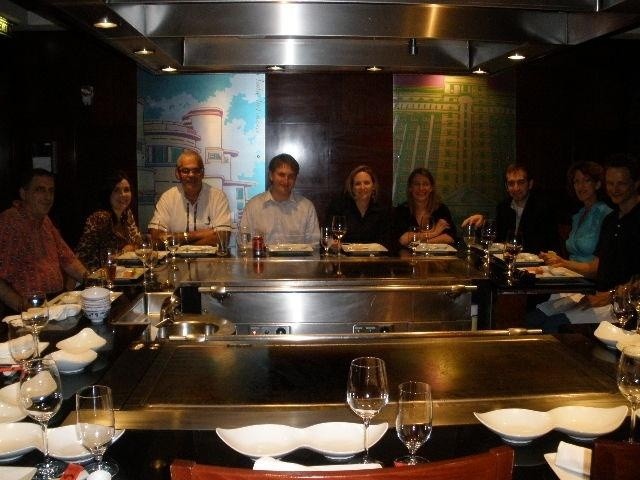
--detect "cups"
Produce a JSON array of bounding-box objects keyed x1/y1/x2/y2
[{"x1": 215, "y1": 226, "x2": 229, "y2": 257}]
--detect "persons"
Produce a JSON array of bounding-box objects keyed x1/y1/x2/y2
[
  {"x1": 564, "y1": 161, "x2": 614, "y2": 295},
  {"x1": 394, "y1": 167, "x2": 458, "y2": 246},
  {"x1": 0, "y1": 168, "x2": 90, "y2": 315},
  {"x1": 330, "y1": 166, "x2": 393, "y2": 244},
  {"x1": 148, "y1": 150, "x2": 231, "y2": 247},
  {"x1": 542, "y1": 163, "x2": 640, "y2": 294},
  {"x1": 66, "y1": 175, "x2": 144, "y2": 291},
  {"x1": 239, "y1": 153, "x2": 321, "y2": 249},
  {"x1": 461, "y1": 161, "x2": 556, "y2": 251}
]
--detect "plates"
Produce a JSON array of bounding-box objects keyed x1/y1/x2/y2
[
  {"x1": 594, "y1": 319, "x2": 640, "y2": 362},
  {"x1": 0, "y1": 466, "x2": 38, "y2": 480},
  {"x1": 267, "y1": 243, "x2": 314, "y2": 256},
  {"x1": 214, "y1": 421, "x2": 388, "y2": 461},
  {"x1": 0, "y1": 330, "x2": 51, "y2": 370},
  {"x1": 171, "y1": 244, "x2": 216, "y2": 257},
  {"x1": 415, "y1": 243, "x2": 458, "y2": 254},
  {"x1": 90, "y1": 266, "x2": 148, "y2": 280},
  {"x1": 473, "y1": 243, "x2": 503, "y2": 253},
  {"x1": 0, "y1": 421, "x2": 126, "y2": 465},
  {"x1": 473, "y1": 404, "x2": 629, "y2": 445},
  {"x1": 49, "y1": 290, "x2": 123, "y2": 310},
  {"x1": 342, "y1": 242, "x2": 388, "y2": 255},
  {"x1": 116, "y1": 251, "x2": 169, "y2": 263},
  {"x1": 492, "y1": 252, "x2": 544, "y2": 264},
  {"x1": 42, "y1": 327, "x2": 108, "y2": 374},
  {"x1": 516, "y1": 265, "x2": 584, "y2": 282}
]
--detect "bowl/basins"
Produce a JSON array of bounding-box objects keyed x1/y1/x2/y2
[{"x1": 80, "y1": 286, "x2": 112, "y2": 325}]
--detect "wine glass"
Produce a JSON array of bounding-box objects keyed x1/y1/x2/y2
[
  {"x1": 165, "y1": 232, "x2": 181, "y2": 272},
  {"x1": 17, "y1": 357, "x2": 67, "y2": 480},
  {"x1": 98, "y1": 247, "x2": 117, "y2": 289},
  {"x1": 158, "y1": 226, "x2": 172, "y2": 266},
  {"x1": 146, "y1": 240, "x2": 160, "y2": 281},
  {"x1": 480, "y1": 219, "x2": 496, "y2": 271},
  {"x1": 319, "y1": 226, "x2": 335, "y2": 259},
  {"x1": 77, "y1": 384, "x2": 120, "y2": 480},
  {"x1": 330, "y1": 215, "x2": 348, "y2": 259},
  {"x1": 408, "y1": 228, "x2": 421, "y2": 258},
  {"x1": 612, "y1": 283, "x2": 631, "y2": 330},
  {"x1": 421, "y1": 215, "x2": 434, "y2": 258},
  {"x1": 237, "y1": 224, "x2": 250, "y2": 250},
  {"x1": 462, "y1": 224, "x2": 476, "y2": 256},
  {"x1": 615, "y1": 345, "x2": 640, "y2": 445},
  {"x1": 345, "y1": 356, "x2": 390, "y2": 464},
  {"x1": 501, "y1": 252, "x2": 519, "y2": 284},
  {"x1": 81, "y1": 267, "x2": 103, "y2": 290},
  {"x1": 234, "y1": 231, "x2": 248, "y2": 259},
  {"x1": 394, "y1": 380, "x2": 435, "y2": 468},
  {"x1": 504, "y1": 236, "x2": 524, "y2": 275},
  {"x1": 20, "y1": 288, "x2": 51, "y2": 358},
  {"x1": 627, "y1": 284, "x2": 640, "y2": 327},
  {"x1": 133, "y1": 234, "x2": 155, "y2": 291},
  {"x1": 5, "y1": 318, "x2": 39, "y2": 373}
]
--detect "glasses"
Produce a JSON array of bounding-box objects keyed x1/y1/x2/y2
[{"x1": 178, "y1": 168, "x2": 206, "y2": 176}]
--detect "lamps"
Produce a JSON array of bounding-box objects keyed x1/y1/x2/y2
[
  {"x1": 135, "y1": 44, "x2": 153, "y2": 56},
  {"x1": 592, "y1": 0, "x2": 602, "y2": 14},
  {"x1": 407, "y1": 39, "x2": 419, "y2": 56},
  {"x1": 94, "y1": 0, "x2": 118, "y2": 29}
]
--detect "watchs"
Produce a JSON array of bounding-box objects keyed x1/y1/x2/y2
[{"x1": 183, "y1": 232, "x2": 188, "y2": 243}]
